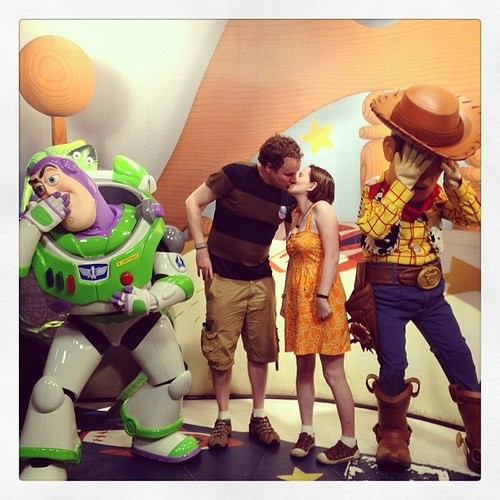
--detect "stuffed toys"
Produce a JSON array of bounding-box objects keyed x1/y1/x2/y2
[{"x1": 21, "y1": 139, "x2": 97, "y2": 215}]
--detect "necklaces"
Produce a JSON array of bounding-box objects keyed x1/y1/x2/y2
[{"x1": 296, "y1": 203, "x2": 315, "y2": 228}]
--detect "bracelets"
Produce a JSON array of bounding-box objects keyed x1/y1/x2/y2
[
  {"x1": 195, "y1": 243, "x2": 207, "y2": 249},
  {"x1": 317, "y1": 292, "x2": 329, "y2": 299}
]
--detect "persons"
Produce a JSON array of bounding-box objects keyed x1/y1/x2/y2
[
  {"x1": 19, "y1": 154, "x2": 195, "y2": 481},
  {"x1": 344, "y1": 84, "x2": 481, "y2": 474},
  {"x1": 280, "y1": 164, "x2": 362, "y2": 464},
  {"x1": 185, "y1": 132, "x2": 303, "y2": 448}
]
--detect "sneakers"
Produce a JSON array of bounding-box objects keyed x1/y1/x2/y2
[
  {"x1": 248, "y1": 414, "x2": 279, "y2": 446},
  {"x1": 317, "y1": 441, "x2": 361, "y2": 465},
  {"x1": 207, "y1": 418, "x2": 231, "y2": 450},
  {"x1": 290, "y1": 431, "x2": 315, "y2": 457}
]
